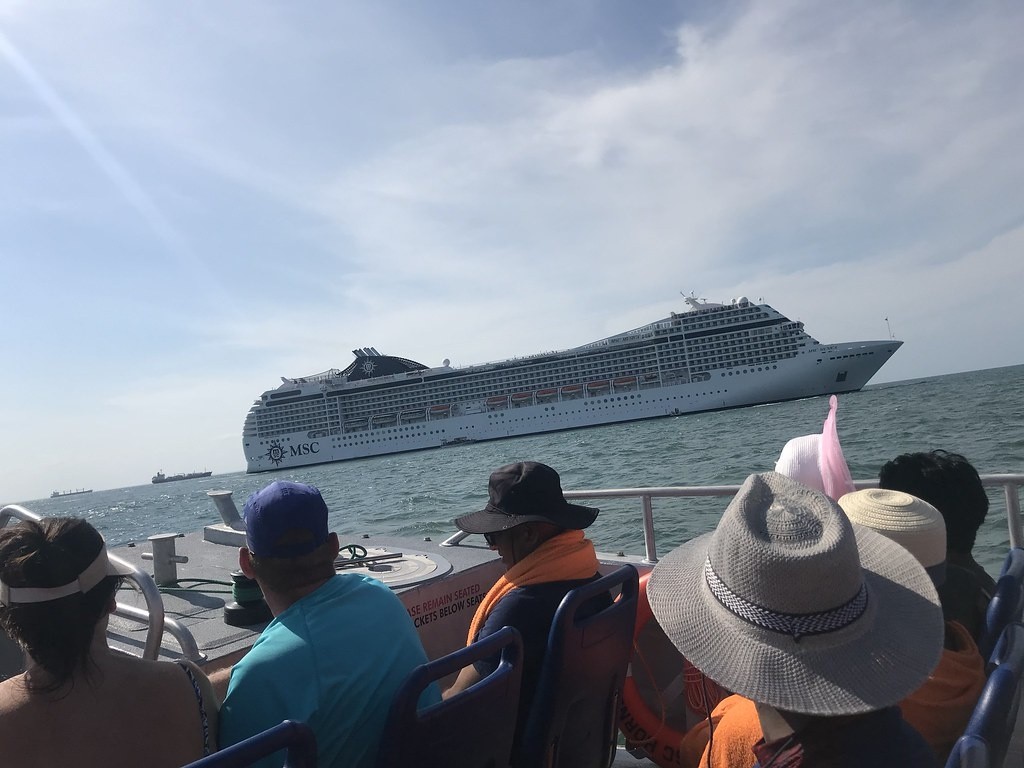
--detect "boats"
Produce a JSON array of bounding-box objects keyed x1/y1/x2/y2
[
  {"x1": 561, "y1": 384, "x2": 583, "y2": 395},
  {"x1": 430, "y1": 405, "x2": 450, "y2": 414},
  {"x1": 486, "y1": 396, "x2": 508, "y2": 405},
  {"x1": 49, "y1": 487, "x2": 94, "y2": 499},
  {"x1": 151, "y1": 469, "x2": 211, "y2": 485},
  {"x1": 535, "y1": 389, "x2": 558, "y2": 398},
  {"x1": 511, "y1": 392, "x2": 532, "y2": 402},
  {"x1": 613, "y1": 376, "x2": 638, "y2": 387},
  {"x1": 587, "y1": 380, "x2": 609, "y2": 392}
]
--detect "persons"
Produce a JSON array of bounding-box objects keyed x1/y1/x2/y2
[
  {"x1": 219, "y1": 480, "x2": 443, "y2": 768},
  {"x1": 645, "y1": 391, "x2": 998, "y2": 767},
  {"x1": 0, "y1": 516, "x2": 219, "y2": 768},
  {"x1": 443, "y1": 460, "x2": 616, "y2": 701}
]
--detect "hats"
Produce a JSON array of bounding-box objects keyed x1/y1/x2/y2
[
  {"x1": 453, "y1": 461, "x2": 599, "y2": 534},
  {"x1": 0, "y1": 532, "x2": 135, "y2": 607},
  {"x1": 775, "y1": 394, "x2": 856, "y2": 502},
  {"x1": 837, "y1": 488, "x2": 954, "y2": 588},
  {"x1": 646, "y1": 471, "x2": 945, "y2": 717},
  {"x1": 242, "y1": 480, "x2": 328, "y2": 558}
]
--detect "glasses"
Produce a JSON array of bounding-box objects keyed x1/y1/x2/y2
[{"x1": 484, "y1": 533, "x2": 500, "y2": 546}]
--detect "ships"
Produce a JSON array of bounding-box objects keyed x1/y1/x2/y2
[{"x1": 238, "y1": 291, "x2": 905, "y2": 475}]
